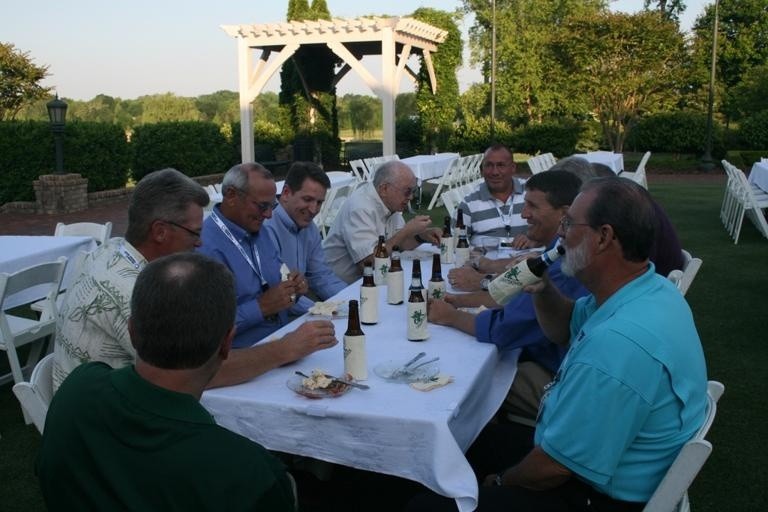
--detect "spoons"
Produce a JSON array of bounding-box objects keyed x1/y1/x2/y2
[{"x1": 325, "y1": 374, "x2": 369, "y2": 391}]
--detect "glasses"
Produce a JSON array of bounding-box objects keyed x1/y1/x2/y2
[
  {"x1": 559, "y1": 214, "x2": 616, "y2": 240},
  {"x1": 251, "y1": 200, "x2": 278, "y2": 212},
  {"x1": 387, "y1": 182, "x2": 418, "y2": 197}
]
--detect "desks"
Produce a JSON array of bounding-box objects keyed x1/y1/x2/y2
[{"x1": 2, "y1": 234, "x2": 95, "y2": 383}]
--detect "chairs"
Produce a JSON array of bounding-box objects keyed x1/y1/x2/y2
[
  {"x1": 640, "y1": 378, "x2": 727, "y2": 512},
  {"x1": 0, "y1": 257, "x2": 70, "y2": 428},
  {"x1": 667, "y1": 249, "x2": 706, "y2": 294},
  {"x1": 30, "y1": 219, "x2": 113, "y2": 316},
  {"x1": 12, "y1": 352, "x2": 55, "y2": 436}
]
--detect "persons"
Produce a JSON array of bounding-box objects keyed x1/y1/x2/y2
[
  {"x1": 424, "y1": 169, "x2": 591, "y2": 419},
  {"x1": 403, "y1": 176, "x2": 712, "y2": 511},
  {"x1": 195, "y1": 162, "x2": 314, "y2": 350},
  {"x1": 447, "y1": 157, "x2": 596, "y2": 289},
  {"x1": 33, "y1": 251, "x2": 296, "y2": 512},
  {"x1": 592, "y1": 162, "x2": 685, "y2": 275},
  {"x1": 324, "y1": 160, "x2": 443, "y2": 285},
  {"x1": 448, "y1": 144, "x2": 540, "y2": 249},
  {"x1": 51, "y1": 166, "x2": 339, "y2": 393},
  {"x1": 272, "y1": 160, "x2": 348, "y2": 315}
]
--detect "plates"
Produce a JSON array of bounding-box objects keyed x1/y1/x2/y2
[
  {"x1": 373, "y1": 355, "x2": 440, "y2": 384},
  {"x1": 289, "y1": 369, "x2": 357, "y2": 399}
]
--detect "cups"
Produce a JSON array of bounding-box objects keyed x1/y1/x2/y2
[
  {"x1": 302, "y1": 315, "x2": 335, "y2": 324},
  {"x1": 480, "y1": 236, "x2": 500, "y2": 258}
]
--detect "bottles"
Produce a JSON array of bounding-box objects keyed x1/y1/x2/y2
[
  {"x1": 407, "y1": 253, "x2": 448, "y2": 341},
  {"x1": 386, "y1": 242, "x2": 405, "y2": 309},
  {"x1": 374, "y1": 233, "x2": 389, "y2": 284},
  {"x1": 440, "y1": 207, "x2": 469, "y2": 270},
  {"x1": 359, "y1": 261, "x2": 377, "y2": 324},
  {"x1": 341, "y1": 298, "x2": 370, "y2": 384},
  {"x1": 488, "y1": 245, "x2": 564, "y2": 307}
]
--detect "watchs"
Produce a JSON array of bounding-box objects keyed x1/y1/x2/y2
[
  {"x1": 480, "y1": 273, "x2": 492, "y2": 292},
  {"x1": 491, "y1": 471, "x2": 504, "y2": 486}
]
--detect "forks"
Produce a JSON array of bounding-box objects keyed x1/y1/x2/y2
[{"x1": 393, "y1": 352, "x2": 441, "y2": 378}]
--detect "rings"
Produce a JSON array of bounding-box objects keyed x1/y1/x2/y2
[
  {"x1": 299, "y1": 280, "x2": 306, "y2": 288},
  {"x1": 450, "y1": 277, "x2": 457, "y2": 286},
  {"x1": 290, "y1": 293, "x2": 296, "y2": 302}
]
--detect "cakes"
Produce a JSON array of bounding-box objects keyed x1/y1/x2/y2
[
  {"x1": 309, "y1": 300, "x2": 344, "y2": 316},
  {"x1": 302, "y1": 369, "x2": 332, "y2": 391}
]
